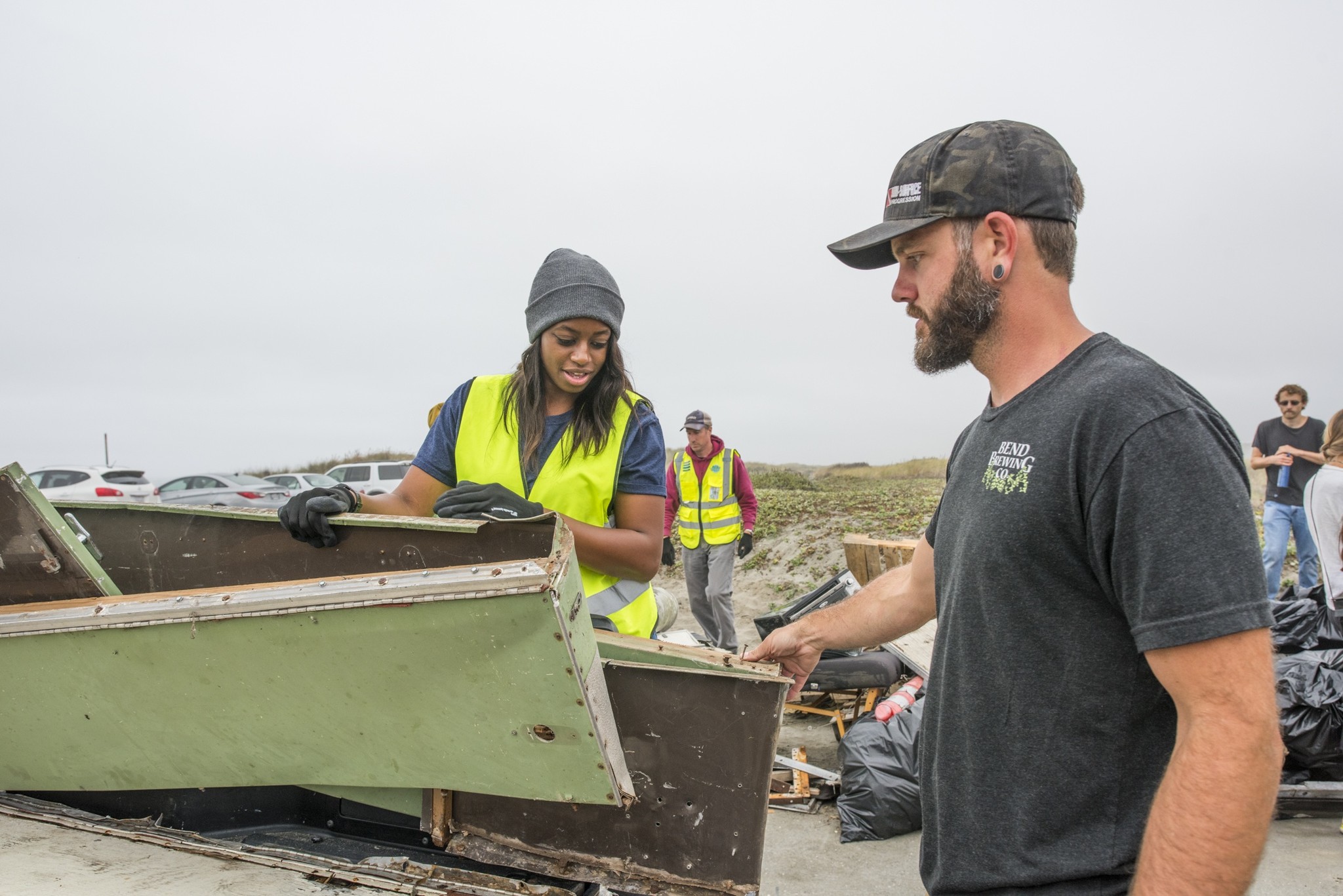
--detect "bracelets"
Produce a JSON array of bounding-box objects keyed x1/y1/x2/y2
[{"x1": 745, "y1": 529, "x2": 754, "y2": 537}]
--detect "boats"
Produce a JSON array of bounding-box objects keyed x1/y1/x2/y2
[{"x1": 0, "y1": 460, "x2": 637, "y2": 815}]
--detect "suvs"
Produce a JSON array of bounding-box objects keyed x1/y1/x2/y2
[{"x1": 325, "y1": 459, "x2": 416, "y2": 495}]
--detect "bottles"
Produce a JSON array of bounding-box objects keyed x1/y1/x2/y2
[{"x1": 1277, "y1": 453, "x2": 1290, "y2": 487}]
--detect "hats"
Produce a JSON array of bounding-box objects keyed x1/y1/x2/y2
[
  {"x1": 679, "y1": 410, "x2": 713, "y2": 431},
  {"x1": 524, "y1": 248, "x2": 625, "y2": 343},
  {"x1": 825, "y1": 119, "x2": 1084, "y2": 272}
]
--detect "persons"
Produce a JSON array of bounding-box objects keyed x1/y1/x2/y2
[
  {"x1": 734, "y1": 117, "x2": 1285, "y2": 895},
  {"x1": 659, "y1": 409, "x2": 759, "y2": 656},
  {"x1": 272, "y1": 246, "x2": 666, "y2": 645},
  {"x1": 1301, "y1": 411, "x2": 1343, "y2": 632},
  {"x1": 1251, "y1": 384, "x2": 1328, "y2": 601}
]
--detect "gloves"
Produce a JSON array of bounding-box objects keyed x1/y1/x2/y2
[
  {"x1": 662, "y1": 537, "x2": 676, "y2": 566},
  {"x1": 276, "y1": 483, "x2": 357, "y2": 550},
  {"x1": 432, "y1": 481, "x2": 544, "y2": 523},
  {"x1": 737, "y1": 533, "x2": 753, "y2": 559}
]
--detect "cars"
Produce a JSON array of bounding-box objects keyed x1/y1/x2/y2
[
  {"x1": 154, "y1": 473, "x2": 292, "y2": 512},
  {"x1": 22, "y1": 465, "x2": 163, "y2": 505},
  {"x1": 259, "y1": 472, "x2": 342, "y2": 495}
]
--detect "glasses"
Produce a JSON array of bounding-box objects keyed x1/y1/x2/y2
[{"x1": 1279, "y1": 400, "x2": 1300, "y2": 405}]
